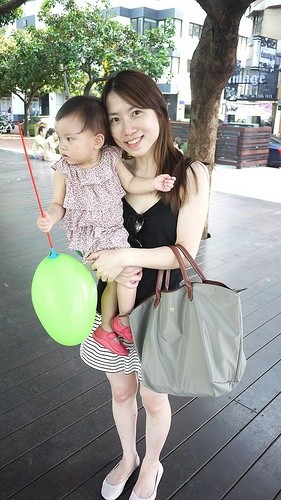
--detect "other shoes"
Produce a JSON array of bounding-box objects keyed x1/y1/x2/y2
[
  {"x1": 129, "y1": 460, "x2": 164, "y2": 500},
  {"x1": 102, "y1": 453, "x2": 140, "y2": 499},
  {"x1": 93, "y1": 326, "x2": 129, "y2": 356},
  {"x1": 111, "y1": 315, "x2": 134, "y2": 343}
]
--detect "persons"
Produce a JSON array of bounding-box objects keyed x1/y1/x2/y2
[
  {"x1": 44, "y1": 127, "x2": 62, "y2": 162},
  {"x1": 36, "y1": 95, "x2": 176, "y2": 357},
  {"x1": 79, "y1": 71, "x2": 211, "y2": 500},
  {"x1": 33, "y1": 125, "x2": 47, "y2": 159}
]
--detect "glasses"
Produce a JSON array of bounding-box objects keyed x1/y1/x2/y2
[{"x1": 126, "y1": 212, "x2": 144, "y2": 249}]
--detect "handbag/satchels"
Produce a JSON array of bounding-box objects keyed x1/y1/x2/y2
[{"x1": 129, "y1": 245, "x2": 247, "y2": 397}]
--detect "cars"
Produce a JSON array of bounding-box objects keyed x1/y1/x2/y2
[
  {"x1": 268, "y1": 135, "x2": 281, "y2": 168},
  {"x1": 20, "y1": 115, "x2": 56, "y2": 138}
]
